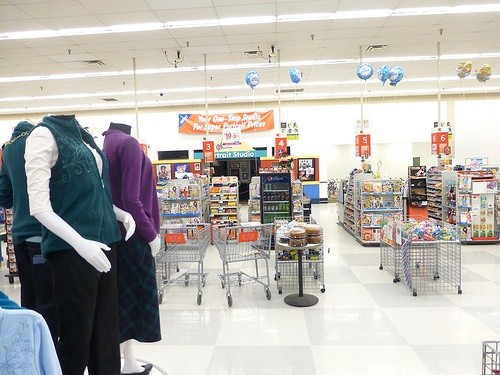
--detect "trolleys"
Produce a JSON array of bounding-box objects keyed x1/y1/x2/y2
[
  {"x1": 212, "y1": 220, "x2": 274, "y2": 307},
  {"x1": 156, "y1": 223, "x2": 212, "y2": 305}
]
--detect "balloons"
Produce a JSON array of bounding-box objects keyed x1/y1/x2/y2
[
  {"x1": 388, "y1": 65, "x2": 404, "y2": 86},
  {"x1": 289, "y1": 67, "x2": 302, "y2": 84},
  {"x1": 356, "y1": 63, "x2": 374, "y2": 81},
  {"x1": 245, "y1": 71, "x2": 260, "y2": 89},
  {"x1": 456, "y1": 61, "x2": 472, "y2": 80},
  {"x1": 377, "y1": 64, "x2": 392, "y2": 85},
  {"x1": 474, "y1": 63, "x2": 492, "y2": 83}
]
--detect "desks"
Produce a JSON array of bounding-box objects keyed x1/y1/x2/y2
[
  {"x1": 278, "y1": 242, "x2": 321, "y2": 307},
  {"x1": 272, "y1": 225, "x2": 326, "y2": 293},
  {"x1": 377, "y1": 229, "x2": 462, "y2": 296}
]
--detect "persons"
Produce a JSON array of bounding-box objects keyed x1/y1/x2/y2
[{"x1": 0, "y1": 115, "x2": 161, "y2": 375}]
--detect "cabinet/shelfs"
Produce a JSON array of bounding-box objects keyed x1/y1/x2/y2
[
  {"x1": 344, "y1": 178, "x2": 406, "y2": 246},
  {"x1": 409, "y1": 166, "x2": 494, "y2": 230},
  {"x1": 160, "y1": 175, "x2": 239, "y2": 242},
  {"x1": 249, "y1": 174, "x2": 306, "y2": 223}
]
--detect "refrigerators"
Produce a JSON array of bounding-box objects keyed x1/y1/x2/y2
[{"x1": 260, "y1": 169, "x2": 294, "y2": 250}]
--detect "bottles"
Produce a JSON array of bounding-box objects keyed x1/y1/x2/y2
[
  {"x1": 264, "y1": 192, "x2": 289, "y2": 201},
  {"x1": 264, "y1": 203, "x2": 289, "y2": 212},
  {"x1": 264, "y1": 214, "x2": 290, "y2": 223}
]
